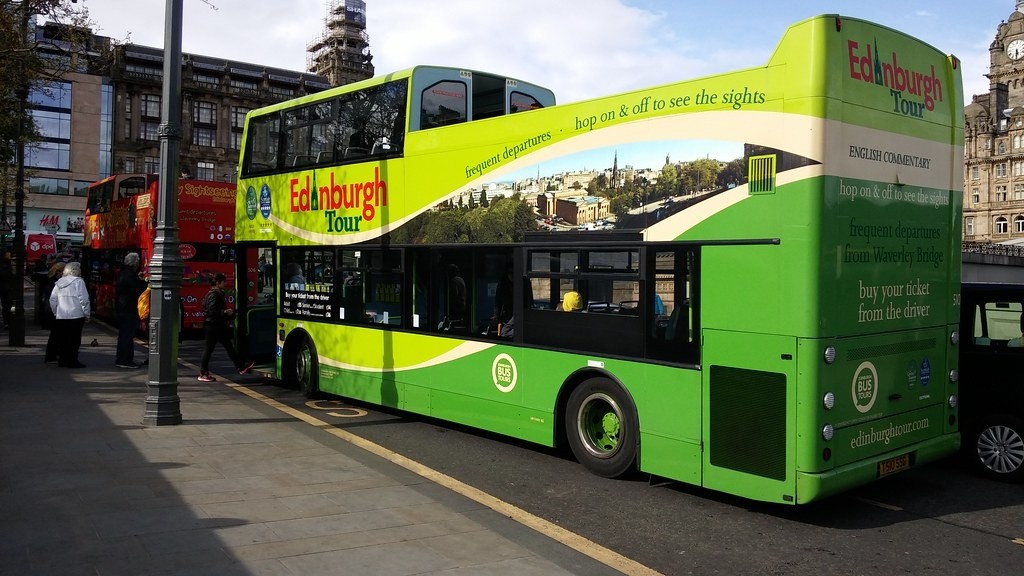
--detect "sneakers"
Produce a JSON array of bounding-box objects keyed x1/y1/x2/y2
[
  {"x1": 239, "y1": 361, "x2": 254, "y2": 376},
  {"x1": 198, "y1": 371, "x2": 215, "y2": 382}
]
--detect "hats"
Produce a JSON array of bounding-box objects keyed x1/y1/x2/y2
[{"x1": 563, "y1": 292, "x2": 584, "y2": 312}]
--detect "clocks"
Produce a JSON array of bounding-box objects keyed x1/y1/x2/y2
[{"x1": 1007, "y1": 39, "x2": 1024, "y2": 60}]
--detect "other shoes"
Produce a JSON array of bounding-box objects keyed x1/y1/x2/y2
[
  {"x1": 59, "y1": 361, "x2": 86, "y2": 367},
  {"x1": 115, "y1": 361, "x2": 140, "y2": 369},
  {"x1": 46, "y1": 357, "x2": 58, "y2": 363}
]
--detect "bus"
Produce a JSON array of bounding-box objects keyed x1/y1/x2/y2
[
  {"x1": 233, "y1": 13, "x2": 965, "y2": 511},
  {"x1": 77, "y1": 173, "x2": 259, "y2": 333}
]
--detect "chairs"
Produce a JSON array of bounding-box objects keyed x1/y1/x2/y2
[
  {"x1": 371, "y1": 143, "x2": 400, "y2": 155},
  {"x1": 587, "y1": 302, "x2": 610, "y2": 313},
  {"x1": 293, "y1": 155, "x2": 317, "y2": 166},
  {"x1": 655, "y1": 315, "x2": 670, "y2": 337},
  {"x1": 343, "y1": 147, "x2": 370, "y2": 159},
  {"x1": 316, "y1": 151, "x2": 333, "y2": 163},
  {"x1": 448, "y1": 320, "x2": 470, "y2": 332},
  {"x1": 248, "y1": 162, "x2": 273, "y2": 173},
  {"x1": 618, "y1": 301, "x2": 639, "y2": 313}
]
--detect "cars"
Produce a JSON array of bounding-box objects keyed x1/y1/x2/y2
[{"x1": 538, "y1": 217, "x2": 614, "y2": 231}]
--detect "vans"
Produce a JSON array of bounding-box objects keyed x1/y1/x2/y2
[{"x1": 23, "y1": 231, "x2": 85, "y2": 276}]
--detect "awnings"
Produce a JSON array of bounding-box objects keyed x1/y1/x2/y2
[{"x1": 55, "y1": 235, "x2": 71, "y2": 240}]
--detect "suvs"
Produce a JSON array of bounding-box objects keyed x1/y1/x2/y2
[{"x1": 960, "y1": 281, "x2": 1024, "y2": 478}]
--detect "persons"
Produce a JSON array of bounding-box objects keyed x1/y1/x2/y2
[
  {"x1": 182, "y1": 168, "x2": 195, "y2": 179},
  {"x1": 448, "y1": 263, "x2": 465, "y2": 310},
  {"x1": 286, "y1": 263, "x2": 307, "y2": 305},
  {"x1": 1007, "y1": 313, "x2": 1024, "y2": 347},
  {"x1": 49, "y1": 261, "x2": 91, "y2": 368},
  {"x1": 349, "y1": 117, "x2": 377, "y2": 154},
  {"x1": 501, "y1": 317, "x2": 515, "y2": 338},
  {"x1": 196, "y1": 272, "x2": 255, "y2": 382},
  {"x1": 257, "y1": 254, "x2": 273, "y2": 287},
  {"x1": 655, "y1": 295, "x2": 664, "y2": 324},
  {"x1": 490, "y1": 263, "x2": 532, "y2": 322},
  {"x1": 556, "y1": 291, "x2": 583, "y2": 311},
  {"x1": 43, "y1": 262, "x2": 65, "y2": 364},
  {"x1": 67, "y1": 217, "x2": 84, "y2": 228},
  {"x1": 115, "y1": 252, "x2": 147, "y2": 368},
  {"x1": 40, "y1": 249, "x2": 81, "y2": 271}
]
